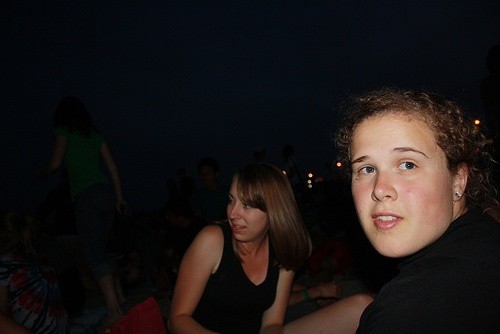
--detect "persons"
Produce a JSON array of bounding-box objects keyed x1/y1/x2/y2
[
  {"x1": 35, "y1": 95, "x2": 129, "y2": 331},
  {"x1": 167, "y1": 164, "x2": 375, "y2": 334},
  {"x1": 0, "y1": 144, "x2": 352, "y2": 334},
  {"x1": 348, "y1": 90, "x2": 500, "y2": 334}
]
